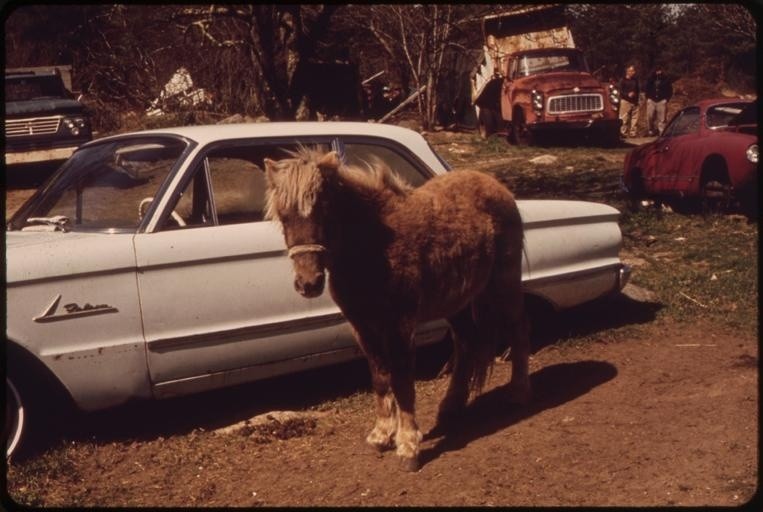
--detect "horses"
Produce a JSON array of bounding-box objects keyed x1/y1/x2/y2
[{"x1": 259, "y1": 138, "x2": 533, "y2": 473}]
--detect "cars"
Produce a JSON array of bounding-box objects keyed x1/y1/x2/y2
[
  {"x1": 622, "y1": 98, "x2": 763, "y2": 209},
  {"x1": 0, "y1": 119, "x2": 630, "y2": 463}
]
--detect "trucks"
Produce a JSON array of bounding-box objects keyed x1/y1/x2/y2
[
  {"x1": 467, "y1": 3, "x2": 622, "y2": 150},
  {"x1": 2, "y1": 63, "x2": 94, "y2": 187}
]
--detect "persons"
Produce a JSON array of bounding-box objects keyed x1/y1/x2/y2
[
  {"x1": 618, "y1": 65, "x2": 640, "y2": 138},
  {"x1": 645, "y1": 65, "x2": 673, "y2": 137}
]
